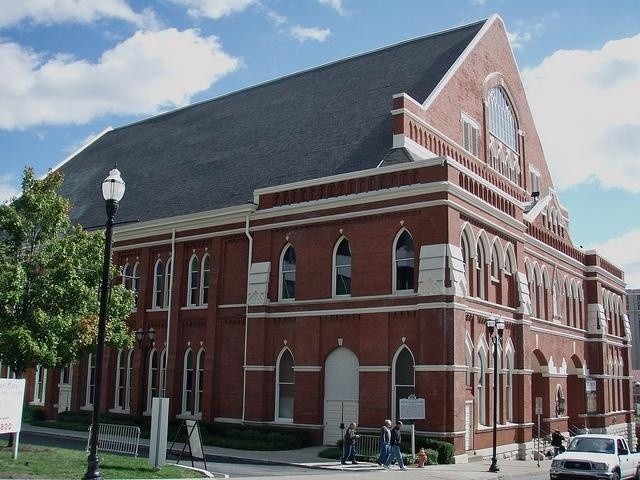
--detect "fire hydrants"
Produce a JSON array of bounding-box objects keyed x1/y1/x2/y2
[{"x1": 417, "y1": 447, "x2": 428, "y2": 468}]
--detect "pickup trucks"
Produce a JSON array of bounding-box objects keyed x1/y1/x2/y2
[{"x1": 550, "y1": 434, "x2": 640, "y2": 480}]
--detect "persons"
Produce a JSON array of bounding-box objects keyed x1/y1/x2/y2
[
  {"x1": 384, "y1": 421, "x2": 408, "y2": 471},
  {"x1": 341, "y1": 422, "x2": 360, "y2": 465},
  {"x1": 550, "y1": 429, "x2": 565, "y2": 457},
  {"x1": 375, "y1": 419, "x2": 392, "y2": 467}
]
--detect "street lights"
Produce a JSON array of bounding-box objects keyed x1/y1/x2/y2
[
  {"x1": 486, "y1": 313, "x2": 506, "y2": 473},
  {"x1": 135, "y1": 325, "x2": 155, "y2": 434},
  {"x1": 82, "y1": 163, "x2": 126, "y2": 480}
]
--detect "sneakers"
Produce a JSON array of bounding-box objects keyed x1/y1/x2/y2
[
  {"x1": 341, "y1": 462, "x2": 346, "y2": 464},
  {"x1": 384, "y1": 465, "x2": 392, "y2": 469},
  {"x1": 400, "y1": 467, "x2": 408, "y2": 470},
  {"x1": 352, "y1": 462, "x2": 358, "y2": 464}
]
